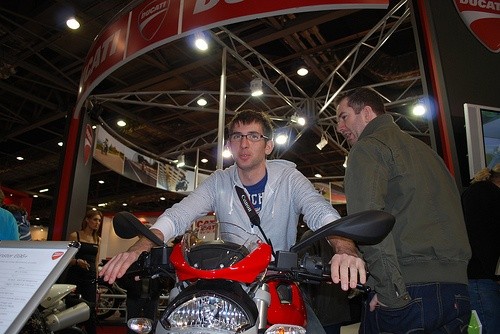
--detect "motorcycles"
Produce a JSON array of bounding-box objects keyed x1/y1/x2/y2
[
  {"x1": 98, "y1": 210, "x2": 395, "y2": 334},
  {"x1": 140, "y1": 165, "x2": 147, "y2": 177},
  {"x1": 95, "y1": 259, "x2": 168, "y2": 322},
  {"x1": 175, "y1": 179, "x2": 189, "y2": 192},
  {"x1": 101, "y1": 141, "x2": 109, "y2": 155}
]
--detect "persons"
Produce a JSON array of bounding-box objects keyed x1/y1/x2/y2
[
  {"x1": 335, "y1": 86, "x2": 476, "y2": 334},
  {"x1": 0, "y1": 209, "x2": 20, "y2": 240},
  {"x1": 99, "y1": 109, "x2": 366, "y2": 334},
  {"x1": 69, "y1": 209, "x2": 103, "y2": 334},
  {"x1": 461, "y1": 162, "x2": 500, "y2": 334}
]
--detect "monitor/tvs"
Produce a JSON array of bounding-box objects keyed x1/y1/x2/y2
[{"x1": 464, "y1": 103, "x2": 500, "y2": 180}]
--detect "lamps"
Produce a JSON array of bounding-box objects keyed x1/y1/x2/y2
[
  {"x1": 250, "y1": 78, "x2": 263, "y2": 97},
  {"x1": 316, "y1": 134, "x2": 328, "y2": 151},
  {"x1": 176, "y1": 155, "x2": 185, "y2": 168}
]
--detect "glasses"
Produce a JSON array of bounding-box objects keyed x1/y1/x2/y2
[{"x1": 230, "y1": 133, "x2": 269, "y2": 141}]
